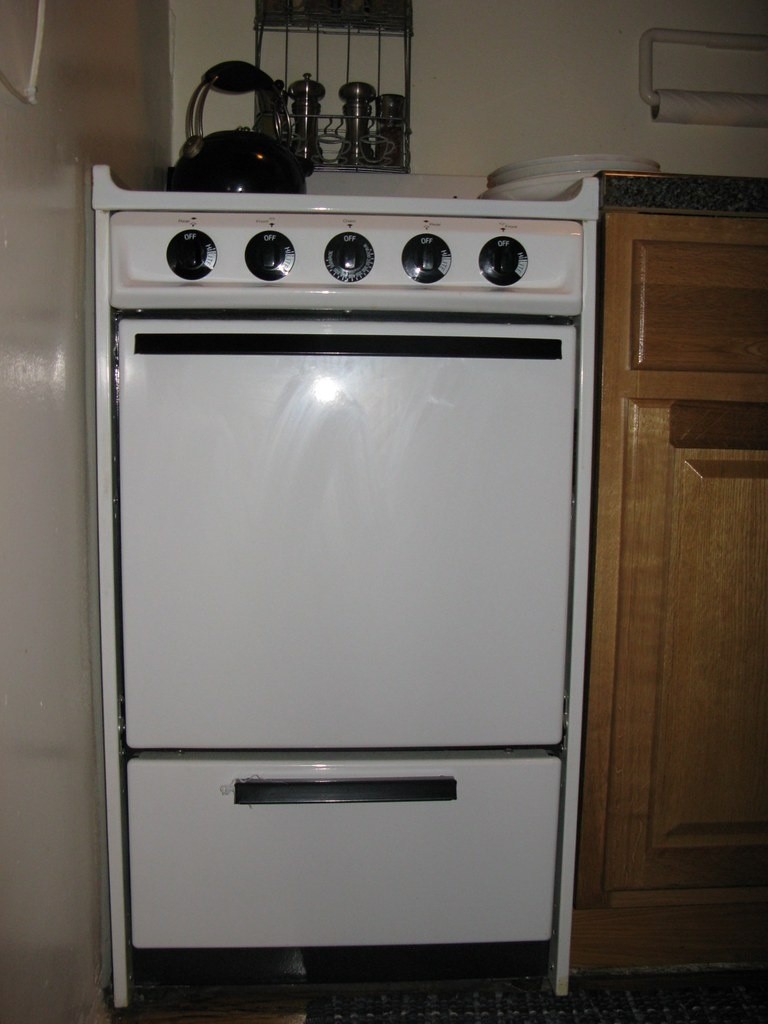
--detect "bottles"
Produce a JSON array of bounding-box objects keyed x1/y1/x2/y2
[
  {"x1": 291, "y1": 73, "x2": 322, "y2": 164},
  {"x1": 337, "y1": 82, "x2": 375, "y2": 164},
  {"x1": 376, "y1": 94, "x2": 404, "y2": 164}
]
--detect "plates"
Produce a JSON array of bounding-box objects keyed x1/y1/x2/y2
[{"x1": 477, "y1": 155, "x2": 661, "y2": 201}]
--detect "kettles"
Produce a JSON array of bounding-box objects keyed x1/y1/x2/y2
[{"x1": 167, "y1": 60, "x2": 316, "y2": 192}]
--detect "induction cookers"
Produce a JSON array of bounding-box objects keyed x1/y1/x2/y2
[{"x1": 88, "y1": 157, "x2": 598, "y2": 309}]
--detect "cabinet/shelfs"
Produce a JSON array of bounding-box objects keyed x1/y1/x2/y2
[{"x1": 571, "y1": 209, "x2": 767, "y2": 969}]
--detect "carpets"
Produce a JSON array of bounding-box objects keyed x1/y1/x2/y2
[{"x1": 304, "y1": 982, "x2": 768, "y2": 1024}]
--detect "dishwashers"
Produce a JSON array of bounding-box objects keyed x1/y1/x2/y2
[{"x1": 117, "y1": 320, "x2": 577, "y2": 949}]
